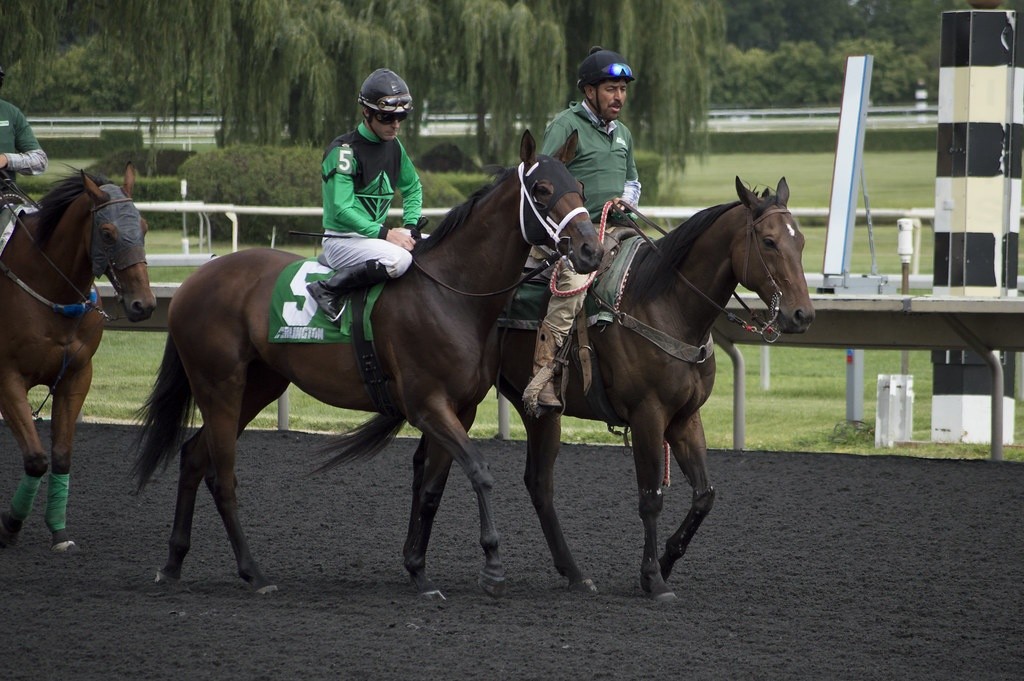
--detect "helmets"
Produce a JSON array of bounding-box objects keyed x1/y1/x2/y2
[
  {"x1": 577, "y1": 46, "x2": 635, "y2": 93},
  {"x1": 358, "y1": 68, "x2": 413, "y2": 113}
]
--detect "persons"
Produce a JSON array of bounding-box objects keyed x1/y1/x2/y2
[
  {"x1": 307, "y1": 68, "x2": 423, "y2": 323},
  {"x1": 528, "y1": 47, "x2": 642, "y2": 408},
  {"x1": 0, "y1": 67, "x2": 51, "y2": 217}
]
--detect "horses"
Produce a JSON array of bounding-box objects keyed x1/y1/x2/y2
[
  {"x1": 401, "y1": 175, "x2": 816, "y2": 602},
  {"x1": 129, "y1": 129, "x2": 604, "y2": 602},
  {"x1": 0, "y1": 161, "x2": 157, "y2": 552}
]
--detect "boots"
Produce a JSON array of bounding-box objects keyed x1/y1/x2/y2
[
  {"x1": 306, "y1": 261, "x2": 368, "y2": 322},
  {"x1": 529, "y1": 322, "x2": 562, "y2": 406}
]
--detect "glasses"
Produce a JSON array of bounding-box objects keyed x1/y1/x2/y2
[
  {"x1": 367, "y1": 106, "x2": 407, "y2": 125},
  {"x1": 361, "y1": 94, "x2": 413, "y2": 112},
  {"x1": 582, "y1": 63, "x2": 632, "y2": 81}
]
